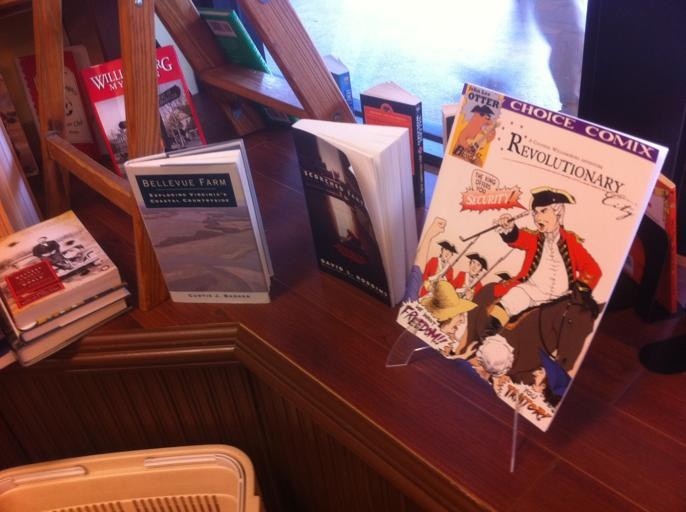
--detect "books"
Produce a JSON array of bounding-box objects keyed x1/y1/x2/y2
[
  {"x1": 395, "y1": 84, "x2": 673, "y2": 434},
  {"x1": 200, "y1": 9, "x2": 296, "y2": 126},
  {"x1": 321, "y1": 52, "x2": 354, "y2": 117},
  {"x1": 291, "y1": 118, "x2": 420, "y2": 306},
  {"x1": 623, "y1": 177, "x2": 679, "y2": 318},
  {"x1": 13, "y1": 43, "x2": 101, "y2": 171},
  {"x1": 75, "y1": 44, "x2": 206, "y2": 180},
  {"x1": 124, "y1": 137, "x2": 275, "y2": 303},
  {"x1": 359, "y1": 82, "x2": 427, "y2": 206},
  {"x1": 0, "y1": 211, "x2": 135, "y2": 371}
]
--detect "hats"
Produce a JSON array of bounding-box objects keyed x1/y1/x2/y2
[
  {"x1": 466, "y1": 252, "x2": 487, "y2": 271},
  {"x1": 495, "y1": 272, "x2": 512, "y2": 281},
  {"x1": 528, "y1": 186, "x2": 576, "y2": 211},
  {"x1": 476, "y1": 334, "x2": 515, "y2": 377},
  {"x1": 437, "y1": 240, "x2": 458, "y2": 254},
  {"x1": 421, "y1": 279, "x2": 478, "y2": 322}
]
